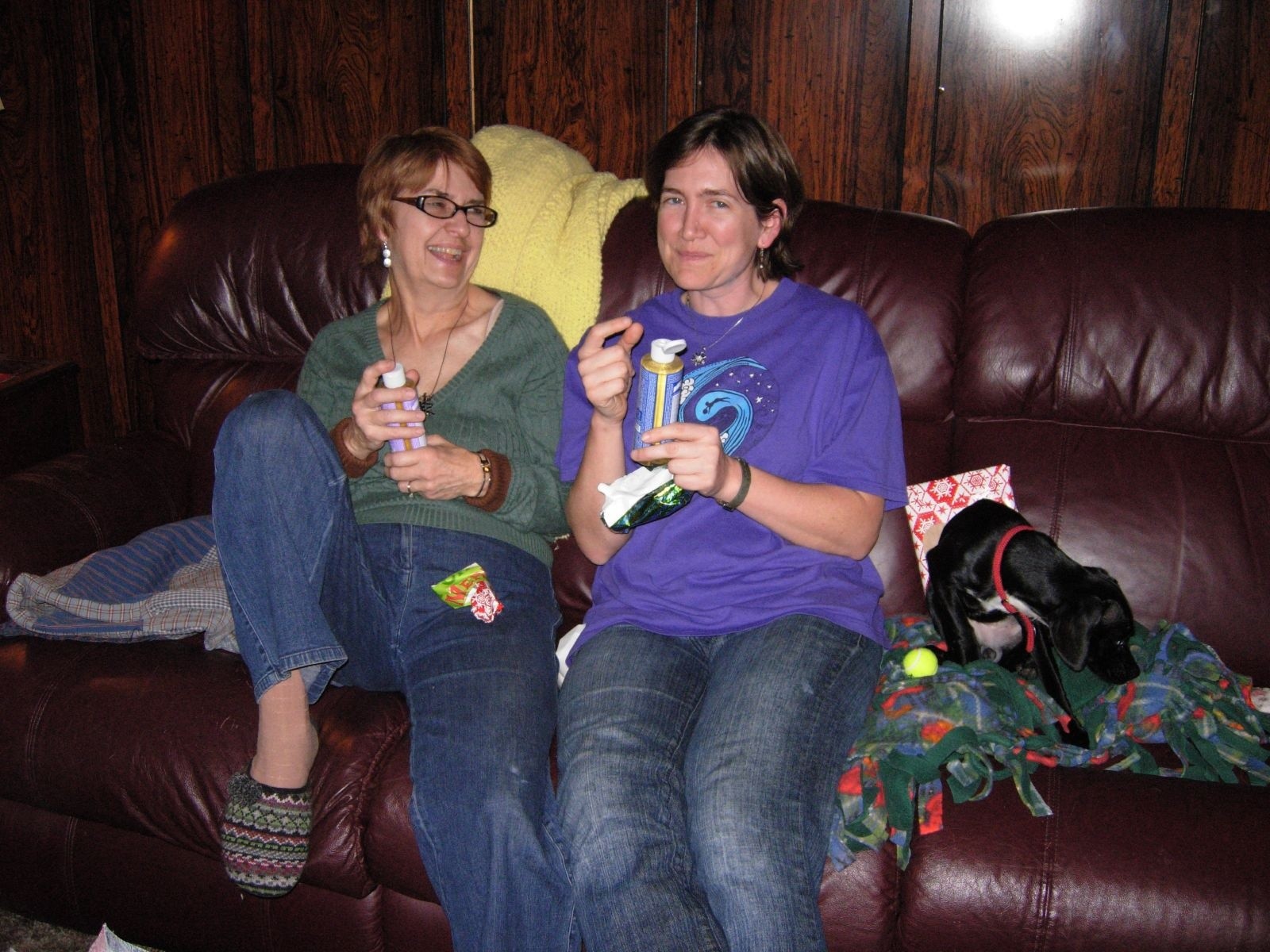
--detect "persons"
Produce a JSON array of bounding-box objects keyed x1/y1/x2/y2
[
  {"x1": 559, "y1": 106, "x2": 908, "y2": 952},
  {"x1": 214, "y1": 128, "x2": 586, "y2": 952}
]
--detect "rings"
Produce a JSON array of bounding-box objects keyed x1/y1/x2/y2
[{"x1": 408, "y1": 480, "x2": 413, "y2": 493}]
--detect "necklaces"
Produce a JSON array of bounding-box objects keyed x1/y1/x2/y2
[
  {"x1": 691, "y1": 281, "x2": 766, "y2": 367},
  {"x1": 386, "y1": 296, "x2": 468, "y2": 418}
]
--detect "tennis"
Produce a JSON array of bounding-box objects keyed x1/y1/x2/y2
[{"x1": 903, "y1": 648, "x2": 940, "y2": 678}]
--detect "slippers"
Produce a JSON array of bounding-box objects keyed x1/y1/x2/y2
[{"x1": 219, "y1": 756, "x2": 311, "y2": 896}]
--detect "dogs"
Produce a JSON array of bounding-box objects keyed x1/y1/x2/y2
[{"x1": 925, "y1": 497, "x2": 1141, "y2": 749}]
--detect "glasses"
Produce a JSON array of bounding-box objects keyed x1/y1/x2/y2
[{"x1": 388, "y1": 194, "x2": 498, "y2": 228}]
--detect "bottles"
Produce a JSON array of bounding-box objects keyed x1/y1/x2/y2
[
  {"x1": 378, "y1": 363, "x2": 426, "y2": 453},
  {"x1": 632, "y1": 338, "x2": 687, "y2": 466}
]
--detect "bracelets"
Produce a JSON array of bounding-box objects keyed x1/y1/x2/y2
[{"x1": 473, "y1": 451, "x2": 492, "y2": 499}]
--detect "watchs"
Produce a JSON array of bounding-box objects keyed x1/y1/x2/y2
[{"x1": 715, "y1": 456, "x2": 752, "y2": 513}]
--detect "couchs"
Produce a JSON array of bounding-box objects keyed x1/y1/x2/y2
[{"x1": 0, "y1": 170, "x2": 1270, "y2": 952}]
